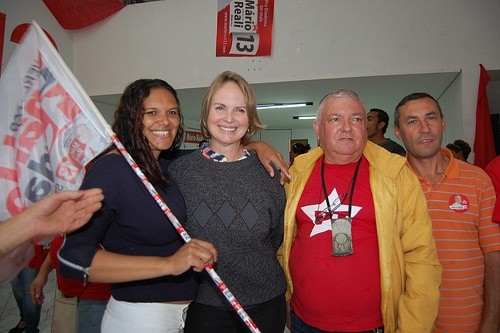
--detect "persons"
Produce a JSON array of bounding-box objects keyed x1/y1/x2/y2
[
  {"x1": 392, "y1": 92, "x2": 500, "y2": 333},
  {"x1": 366, "y1": 108, "x2": 406, "y2": 157},
  {"x1": 289, "y1": 141, "x2": 311, "y2": 163},
  {"x1": 446, "y1": 140, "x2": 471, "y2": 163},
  {"x1": 276, "y1": 89, "x2": 442, "y2": 333},
  {"x1": 58, "y1": 78, "x2": 291, "y2": 333},
  {"x1": 31, "y1": 234, "x2": 112, "y2": 333},
  {"x1": 8, "y1": 243, "x2": 53, "y2": 333},
  {"x1": 166, "y1": 71, "x2": 288, "y2": 332},
  {"x1": 0, "y1": 188, "x2": 104, "y2": 258}
]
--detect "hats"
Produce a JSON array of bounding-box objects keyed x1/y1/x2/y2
[{"x1": 445, "y1": 140, "x2": 471, "y2": 155}]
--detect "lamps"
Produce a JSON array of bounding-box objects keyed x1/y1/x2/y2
[
  {"x1": 256, "y1": 102, "x2": 313, "y2": 110},
  {"x1": 293, "y1": 115, "x2": 316, "y2": 120}
]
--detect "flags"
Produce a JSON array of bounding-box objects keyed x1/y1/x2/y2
[{"x1": 0, "y1": 24, "x2": 113, "y2": 221}]
